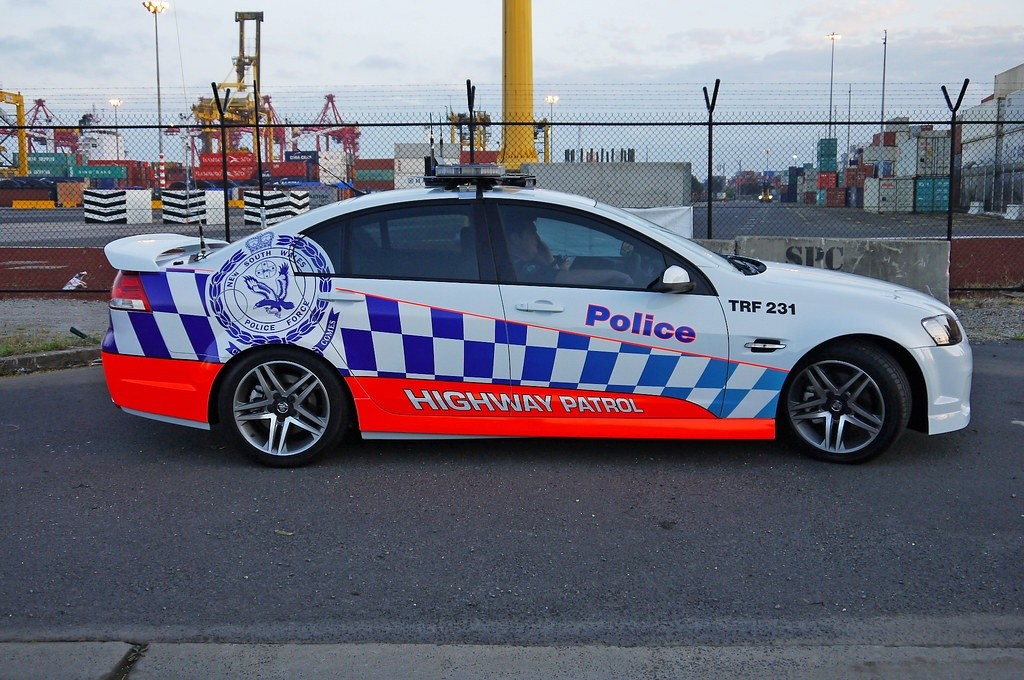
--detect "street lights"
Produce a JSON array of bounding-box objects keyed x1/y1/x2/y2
[
  {"x1": 142, "y1": 0, "x2": 166, "y2": 194},
  {"x1": 109, "y1": 98, "x2": 123, "y2": 167}
]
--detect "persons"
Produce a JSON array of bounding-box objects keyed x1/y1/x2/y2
[{"x1": 506, "y1": 212, "x2": 635, "y2": 288}]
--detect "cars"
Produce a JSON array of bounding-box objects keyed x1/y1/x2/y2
[{"x1": 98, "y1": 164, "x2": 980, "y2": 465}]
